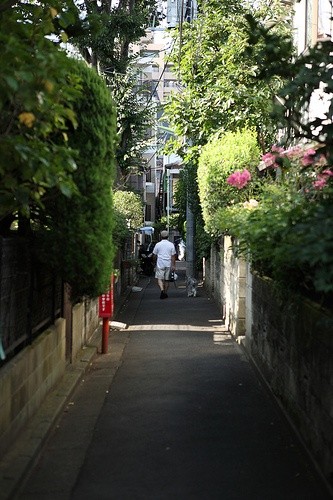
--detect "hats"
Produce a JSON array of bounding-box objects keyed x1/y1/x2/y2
[{"x1": 161, "y1": 231, "x2": 169, "y2": 237}]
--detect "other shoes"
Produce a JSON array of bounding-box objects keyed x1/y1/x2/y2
[{"x1": 160, "y1": 290, "x2": 168, "y2": 299}]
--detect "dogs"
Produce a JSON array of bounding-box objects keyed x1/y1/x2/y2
[{"x1": 184, "y1": 275, "x2": 197, "y2": 297}]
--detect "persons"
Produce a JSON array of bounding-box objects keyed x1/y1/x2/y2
[
  {"x1": 151, "y1": 230, "x2": 176, "y2": 299},
  {"x1": 138, "y1": 235, "x2": 185, "y2": 275}
]
